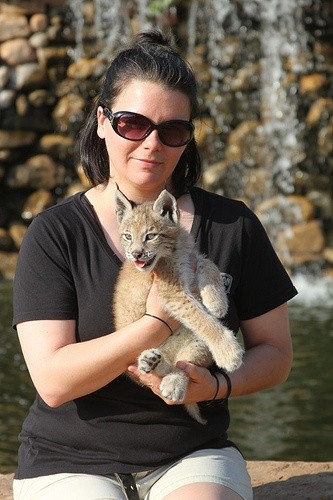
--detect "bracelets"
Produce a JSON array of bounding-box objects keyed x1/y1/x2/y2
[
  {"x1": 204, "y1": 369, "x2": 231, "y2": 407},
  {"x1": 144, "y1": 313, "x2": 173, "y2": 336}
]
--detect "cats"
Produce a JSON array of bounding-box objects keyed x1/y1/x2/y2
[{"x1": 106, "y1": 182, "x2": 250, "y2": 425}]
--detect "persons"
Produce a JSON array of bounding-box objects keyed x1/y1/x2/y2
[{"x1": 12, "y1": 30, "x2": 298, "y2": 500}]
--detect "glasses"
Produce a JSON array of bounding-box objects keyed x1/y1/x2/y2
[{"x1": 100, "y1": 102, "x2": 194, "y2": 150}]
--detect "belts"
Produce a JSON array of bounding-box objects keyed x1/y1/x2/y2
[{"x1": 116, "y1": 468, "x2": 140, "y2": 500}]
